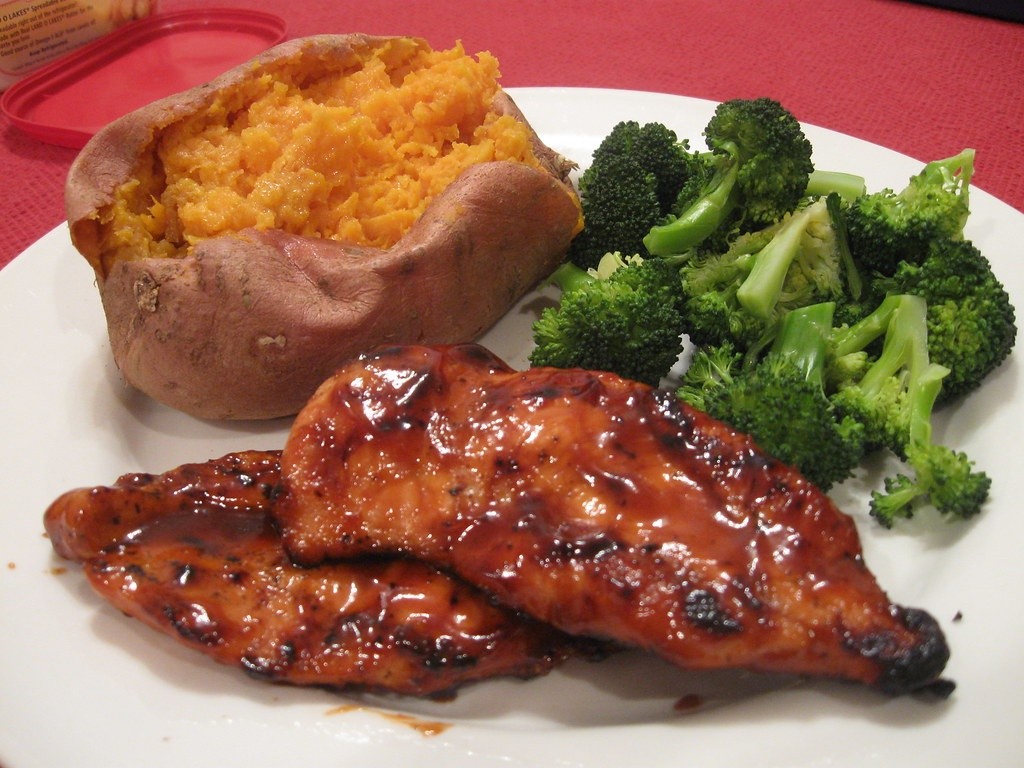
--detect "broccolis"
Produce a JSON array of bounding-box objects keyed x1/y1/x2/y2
[{"x1": 524, "y1": 98, "x2": 1018, "y2": 527}]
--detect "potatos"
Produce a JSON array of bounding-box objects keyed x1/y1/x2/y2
[{"x1": 66, "y1": 32, "x2": 585, "y2": 421}]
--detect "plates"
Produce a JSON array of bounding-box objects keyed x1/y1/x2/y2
[{"x1": 1, "y1": 86, "x2": 1024, "y2": 768}]
